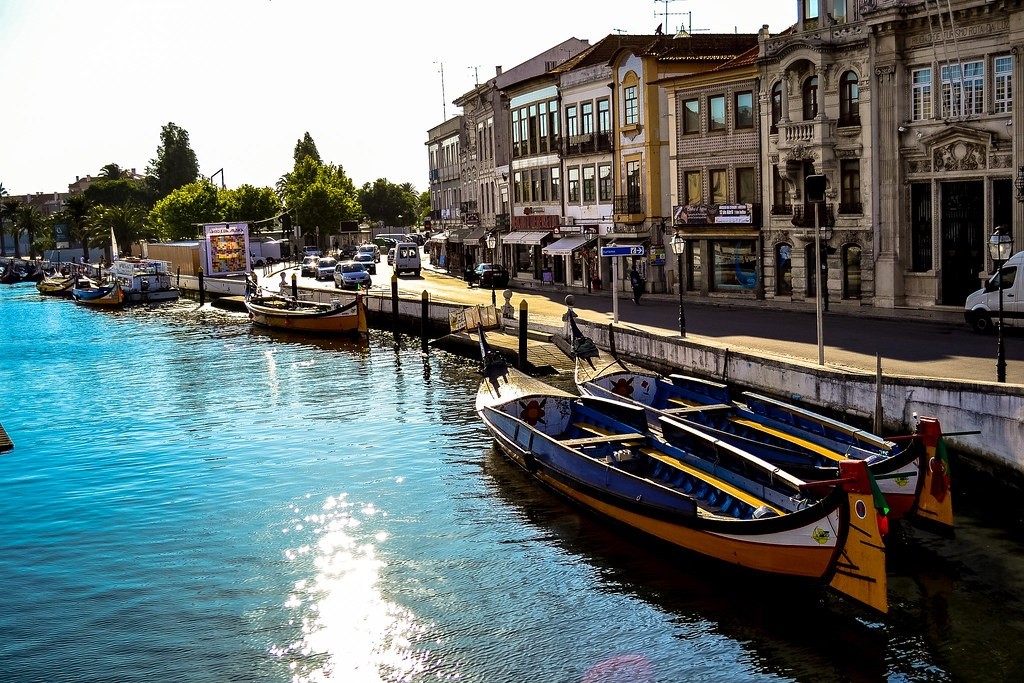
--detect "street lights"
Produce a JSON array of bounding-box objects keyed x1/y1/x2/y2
[
  {"x1": 669, "y1": 231, "x2": 688, "y2": 337},
  {"x1": 986, "y1": 224, "x2": 1015, "y2": 383},
  {"x1": 485, "y1": 233, "x2": 497, "y2": 305}
]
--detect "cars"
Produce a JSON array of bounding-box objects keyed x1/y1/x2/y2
[
  {"x1": 302, "y1": 246, "x2": 323, "y2": 259},
  {"x1": 340, "y1": 246, "x2": 359, "y2": 260},
  {"x1": 250, "y1": 254, "x2": 267, "y2": 266},
  {"x1": 377, "y1": 234, "x2": 425, "y2": 248},
  {"x1": 352, "y1": 254, "x2": 376, "y2": 274},
  {"x1": 465, "y1": 263, "x2": 510, "y2": 287},
  {"x1": 424, "y1": 240, "x2": 431, "y2": 253}
]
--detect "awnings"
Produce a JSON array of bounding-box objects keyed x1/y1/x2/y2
[
  {"x1": 431, "y1": 230, "x2": 452, "y2": 244},
  {"x1": 448, "y1": 228, "x2": 472, "y2": 245},
  {"x1": 463, "y1": 226, "x2": 496, "y2": 246},
  {"x1": 542, "y1": 234, "x2": 599, "y2": 257},
  {"x1": 502, "y1": 230, "x2": 550, "y2": 245}
]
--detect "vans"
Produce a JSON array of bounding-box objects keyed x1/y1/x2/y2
[
  {"x1": 393, "y1": 243, "x2": 421, "y2": 276},
  {"x1": 964, "y1": 251, "x2": 1024, "y2": 333}
]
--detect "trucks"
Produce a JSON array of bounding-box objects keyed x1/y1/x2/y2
[{"x1": 250, "y1": 237, "x2": 291, "y2": 264}]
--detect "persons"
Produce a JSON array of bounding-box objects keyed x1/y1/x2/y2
[
  {"x1": 629, "y1": 265, "x2": 644, "y2": 304},
  {"x1": 332, "y1": 237, "x2": 339, "y2": 252},
  {"x1": 80, "y1": 251, "x2": 130, "y2": 270}
]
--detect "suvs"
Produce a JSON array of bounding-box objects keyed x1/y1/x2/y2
[
  {"x1": 387, "y1": 248, "x2": 395, "y2": 265},
  {"x1": 357, "y1": 245, "x2": 380, "y2": 263},
  {"x1": 333, "y1": 262, "x2": 372, "y2": 288},
  {"x1": 301, "y1": 256, "x2": 320, "y2": 276},
  {"x1": 314, "y1": 257, "x2": 338, "y2": 280}
]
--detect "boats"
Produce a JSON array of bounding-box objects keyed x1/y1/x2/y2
[
  {"x1": 475, "y1": 322, "x2": 895, "y2": 616},
  {"x1": 36, "y1": 270, "x2": 74, "y2": 295},
  {"x1": 0, "y1": 259, "x2": 50, "y2": 282},
  {"x1": 244, "y1": 279, "x2": 368, "y2": 334},
  {"x1": 567, "y1": 311, "x2": 955, "y2": 532},
  {"x1": 71, "y1": 269, "x2": 123, "y2": 305},
  {"x1": 105, "y1": 257, "x2": 180, "y2": 300}
]
